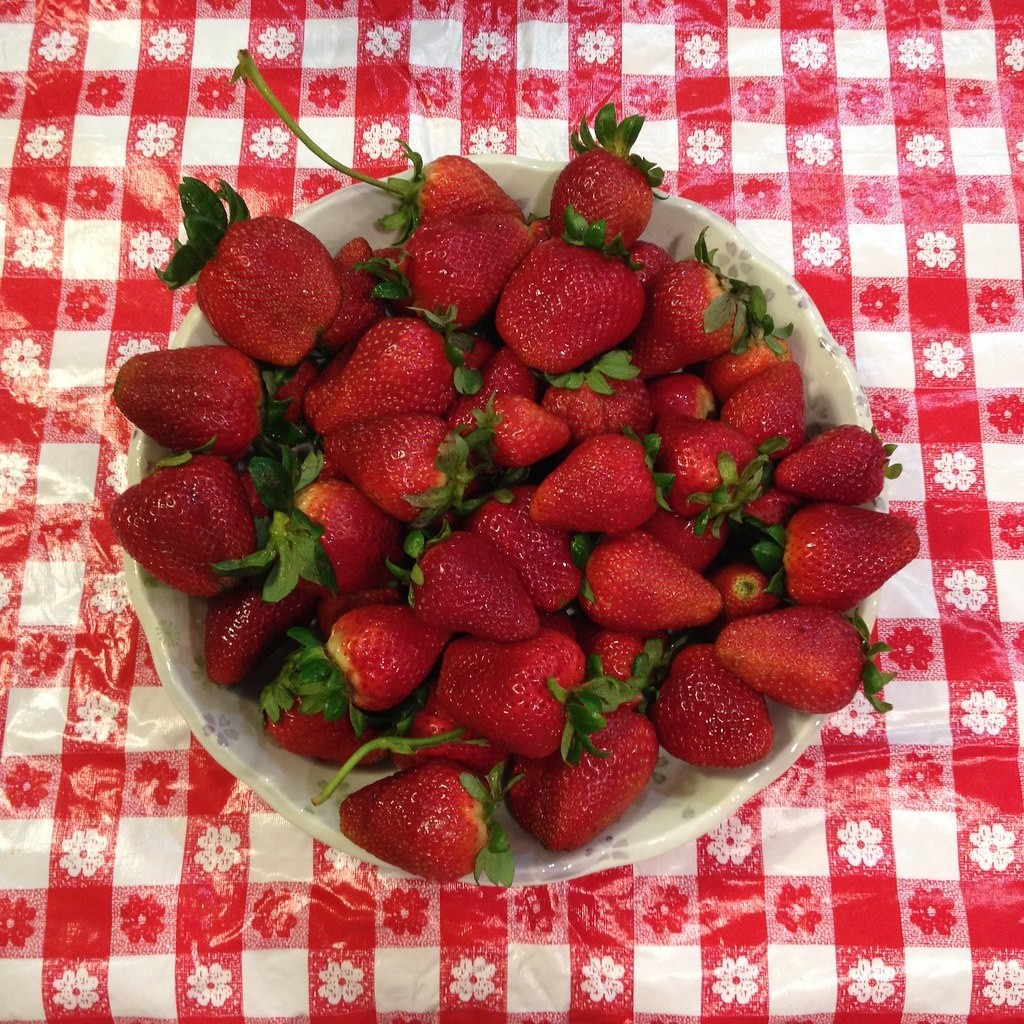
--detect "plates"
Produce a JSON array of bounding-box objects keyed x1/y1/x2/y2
[{"x1": 122, "y1": 154, "x2": 889, "y2": 887}]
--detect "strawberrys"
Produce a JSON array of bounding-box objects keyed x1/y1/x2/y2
[{"x1": 105, "y1": 101, "x2": 920, "y2": 889}]
